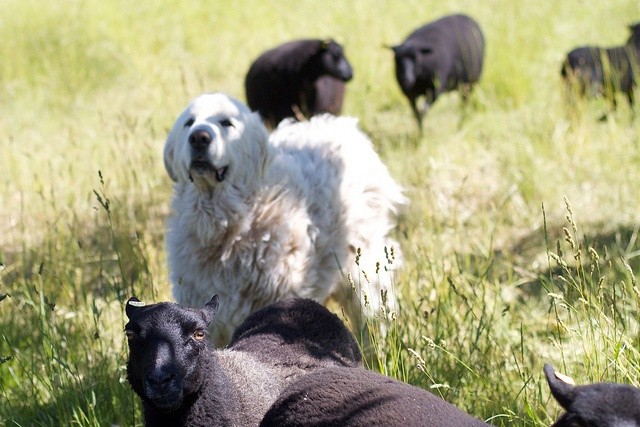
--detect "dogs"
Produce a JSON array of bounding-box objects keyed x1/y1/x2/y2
[{"x1": 161, "y1": 91, "x2": 413, "y2": 357}]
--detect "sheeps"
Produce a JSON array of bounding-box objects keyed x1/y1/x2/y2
[
  {"x1": 385, "y1": 12, "x2": 488, "y2": 138},
  {"x1": 560, "y1": 23, "x2": 640, "y2": 126},
  {"x1": 243, "y1": 35, "x2": 357, "y2": 124},
  {"x1": 258, "y1": 364, "x2": 640, "y2": 427},
  {"x1": 122, "y1": 292, "x2": 366, "y2": 427}
]
